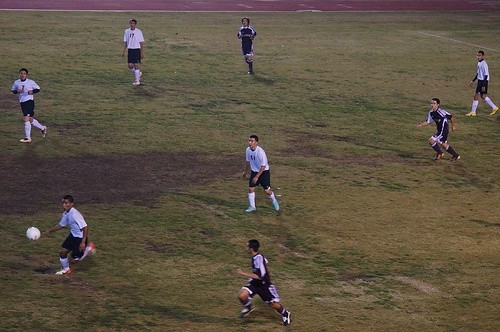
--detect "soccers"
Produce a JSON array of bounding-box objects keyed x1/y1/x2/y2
[{"x1": 26, "y1": 227, "x2": 41, "y2": 241}]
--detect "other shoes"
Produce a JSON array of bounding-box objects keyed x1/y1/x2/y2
[{"x1": 247, "y1": 70, "x2": 257, "y2": 75}]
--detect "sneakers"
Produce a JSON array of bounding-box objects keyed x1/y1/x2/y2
[
  {"x1": 89, "y1": 242, "x2": 97, "y2": 255},
  {"x1": 283, "y1": 309, "x2": 291, "y2": 325},
  {"x1": 465, "y1": 112, "x2": 477, "y2": 117},
  {"x1": 488, "y1": 106, "x2": 499, "y2": 116},
  {"x1": 240, "y1": 303, "x2": 257, "y2": 317},
  {"x1": 133, "y1": 71, "x2": 142, "y2": 86},
  {"x1": 41, "y1": 125, "x2": 48, "y2": 138},
  {"x1": 448, "y1": 154, "x2": 461, "y2": 162},
  {"x1": 55, "y1": 268, "x2": 74, "y2": 276},
  {"x1": 19, "y1": 137, "x2": 33, "y2": 143},
  {"x1": 272, "y1": 199, "x2": 280, "y2": 211},
  {"x1": 245, "y1": 205, "x2": 258, "y2": 213},
  {"x1": 434, "y1": 151, "x2": 446, "y2": 160}
]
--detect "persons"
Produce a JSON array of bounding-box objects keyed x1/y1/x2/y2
[
  {"x1": 43, "y1": 193, "x2": 96, "y2": 275},
  {"x1": 464, "y1": 50, "x2": 499, "y2": 116},
  {"x1": 241, "y1": 134, "x2": 280, "y2": 213},
  {"x1": 121, "y1": 18, "x2": 144, "y2": 86},
  {"x1": 236, "y1": 16, "x2": 257, "y2": 74},
  {"x1": 235, "y1": 239, "x2": 292, "y2": 326},
  {"x1": 11, "y1": 66, "x2": 47, "y2": 144},
  {"x1": 416, "y1": 97, "x2": 461, "y2": 160}
]
